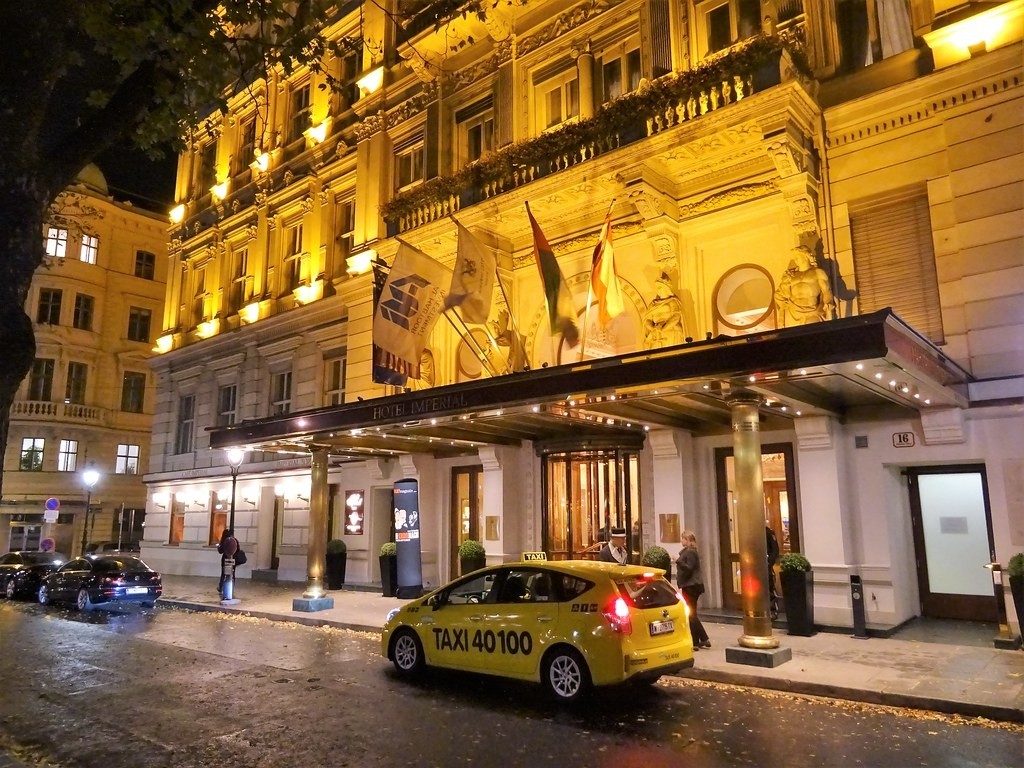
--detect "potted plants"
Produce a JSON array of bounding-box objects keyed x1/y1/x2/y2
[
  {"x1": 324, "y1": 538, "x2": 346, "y2": 590},
  {"x1": 1008, "y1": 552, "x2": 1024, "y2": 636},
  {"x1": 459, "y1": 539, "x2": 488, "y2": 574},
  {"x1": 645, "y1": 546, "x2": 672, "y2": 584},
  {"x1": 378, "y1": 541, "x2": 397, "y2": 597},
  {"x1": 780, "y1": 552, "x2": 818, "y2": 637}
]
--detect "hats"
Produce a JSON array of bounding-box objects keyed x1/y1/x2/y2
[{"x1": 611, "y1": 528, "x2": 626, "y2": 537}]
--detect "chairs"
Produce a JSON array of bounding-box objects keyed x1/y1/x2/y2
[
  {"x1": 504, "y1": 577, "x2": 526, "y2": 603},
  {"x1": 535, "y1": 577, "x2": 551, "y2": 600}
]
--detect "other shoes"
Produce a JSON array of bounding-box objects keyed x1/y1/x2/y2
[
  {"x1": 692, "y1": 646, "x2": 698, "y2": 651},
  {"x1": 699, "y1": 641, "x2": 711, "y2": 647},
  {"x1": 217, "y1": 587, "x2": 221, "y2": 592}
]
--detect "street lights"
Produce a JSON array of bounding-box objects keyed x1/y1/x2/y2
[
  {"x1": 226, "y1": 449, "x2": 244, "y2": 600},
  {"x1": 80, "y1": 470, "x2": 98, "y2": 555}
]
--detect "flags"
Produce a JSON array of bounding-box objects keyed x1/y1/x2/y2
[{"x1": 372, "y1": 200, "x2": 625, "y2": 388}]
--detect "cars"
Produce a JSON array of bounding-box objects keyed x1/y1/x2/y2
[
  {"x1": 82, "y1": 541, "x2": 140, "y2": 558},
  {"x1": 0, "y1": 551, "x2": 63, "y2": 598},
  {"x1": 39, "y1": 555, "x2": 163, "y2": 613},
  {"x1": 382, "y1": 551, "x2": 695, "y2": 711}
]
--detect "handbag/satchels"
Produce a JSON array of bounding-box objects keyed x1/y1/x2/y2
[{"x1": 234, "y1": 550, "x2": 246, "y2": 565}]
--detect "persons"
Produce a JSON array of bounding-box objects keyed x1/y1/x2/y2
[
  {"x1": 216, "y1": 530, "x2": 240, "y2": 593},
  {"x1": 676, "y1": 531, "x2": 711, "y2": 650},
  {"x1": 641, "y1": 275, "x2": 684, "y2": 351},
  {"x1": 774, "y1": 246, "x2": 834, "y2": 330},
  {"x1": 481, "y1": 321, "x2": 518, "y2": 380},
  {"x1": 765, "y1": 526, "x2": 780, "y2": 619},
  {"x1": 408, "y1": 347, "x2": 435, "y2": 391},
  {"x1": 600, "y1": 528, "x2": 628, "y2": 564}
]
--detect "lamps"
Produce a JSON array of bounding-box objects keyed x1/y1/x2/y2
[
  {"x1": 542, "y1": 362, "x2": 548, "y2": 368},
  {"x1": 523, "y1": 365, "x2": 530, "y2": 373},
  {"x1": 357, "y1": 396, "x2": 363, "y2": 401},
  {"x1": 706, "y1": 332, "x2": 712, "y2": 339},
  {"x1": 686, "y1": 337, "x2": 693, "y2": 343}
]
